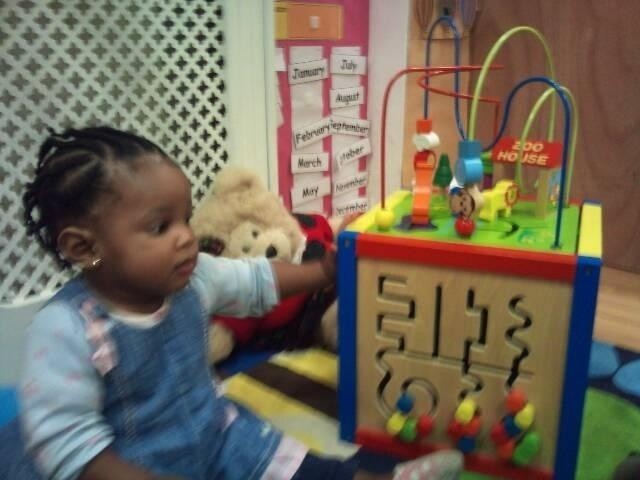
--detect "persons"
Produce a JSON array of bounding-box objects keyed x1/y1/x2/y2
[{"x1": 16, "y1": 121, "x2": 466, "y2": 480}]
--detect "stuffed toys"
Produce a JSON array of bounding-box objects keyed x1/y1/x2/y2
[{"x1": 186, "y1": 162, "x2": 361, "y2": 366}]
